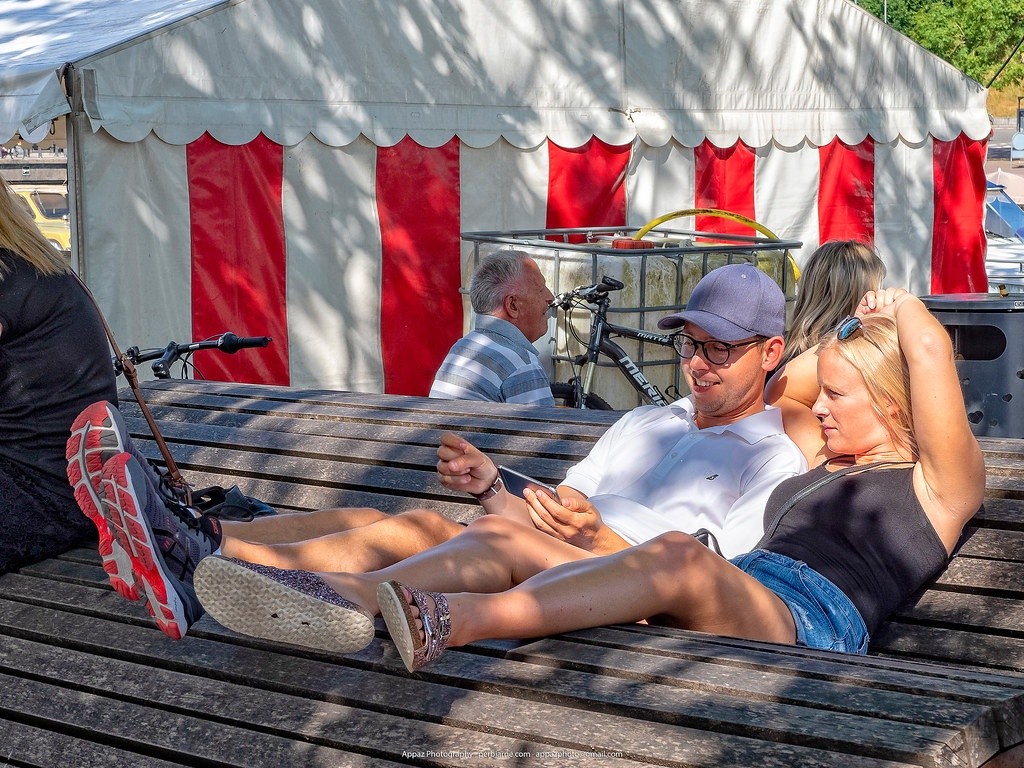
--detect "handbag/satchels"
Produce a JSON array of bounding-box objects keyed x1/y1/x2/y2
[{"x1": 152, "y1": 464, "x2": 192, "y2": 496}]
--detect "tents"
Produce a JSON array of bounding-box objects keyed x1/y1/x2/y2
[{"x1": 0, "y1": 1, "x2": 993, "y2": 395}]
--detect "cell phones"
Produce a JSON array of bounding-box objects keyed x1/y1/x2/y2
[{"x1": 498, "y1": 464, "x2": 562, "y2": 505}]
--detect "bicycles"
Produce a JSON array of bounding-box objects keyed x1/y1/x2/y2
[
  {"x1": 4, "y1": 143, "x2": 25, "y2": 160},
  {"x1": 549, "y1": 274, "x2": 683, "y2": 410},
  {"x1": 114, "y1": 332, "x2": 272, "y2": 380}
]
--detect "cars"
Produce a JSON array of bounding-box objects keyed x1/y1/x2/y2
[{"x1": 17, "y1": 188, "x2": 71, "y2": 252}]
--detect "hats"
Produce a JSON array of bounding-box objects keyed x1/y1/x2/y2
[{"x1": 657, "y1": 262, "x2": 786, "y2": 342}]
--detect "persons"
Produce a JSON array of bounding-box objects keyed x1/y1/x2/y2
[
  {"x1": 429, "y1": 251, "x2": 558, "y2": 407},
  {"x1": 191, "y1": 288, "x2": 986, "y2": 672},
  {"x1": 66, "y1": 263, "x2": 808, "y2": 640},
  {"x1": 0, "y1": 172, "x2": 118, "y2": 571},
  {"x1": 765, "y1": 240, "x2": 886, "y2": 387}
]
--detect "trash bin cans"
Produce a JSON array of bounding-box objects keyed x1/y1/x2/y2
[
  {"x1": 1015, "y1": 96, "x2": 1024, "y2": 133},
  {"x1": 918, "y1": 293, "x2": 1024, "y2": 437}
]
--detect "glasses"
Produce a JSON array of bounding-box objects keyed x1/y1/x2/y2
[
  {"x1": 835, "y1": 314, "x2": 882, "y2": 353},
  {"x1": 669, "y1": 329, "x2": 766, "y2": 366}
]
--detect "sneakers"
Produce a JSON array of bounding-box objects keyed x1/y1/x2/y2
[
  {"x1": 64, "y1": 399, "x2": 187, "y2": 601},
  {"x1": 99, "y1": 452, "x2": 222, "y2": 640}
]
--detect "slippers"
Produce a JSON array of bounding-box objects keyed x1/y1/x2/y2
[
  {"x1": 376, "y1": 578, "x2": 453, "y2": 673},
  {"x1": 192, "y1": 553, "x2": 376, "y2": 654}
]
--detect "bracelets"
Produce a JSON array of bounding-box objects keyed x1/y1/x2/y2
[{"x1": 468, "y1": 472, "x2": 504, "y2": 501}]
